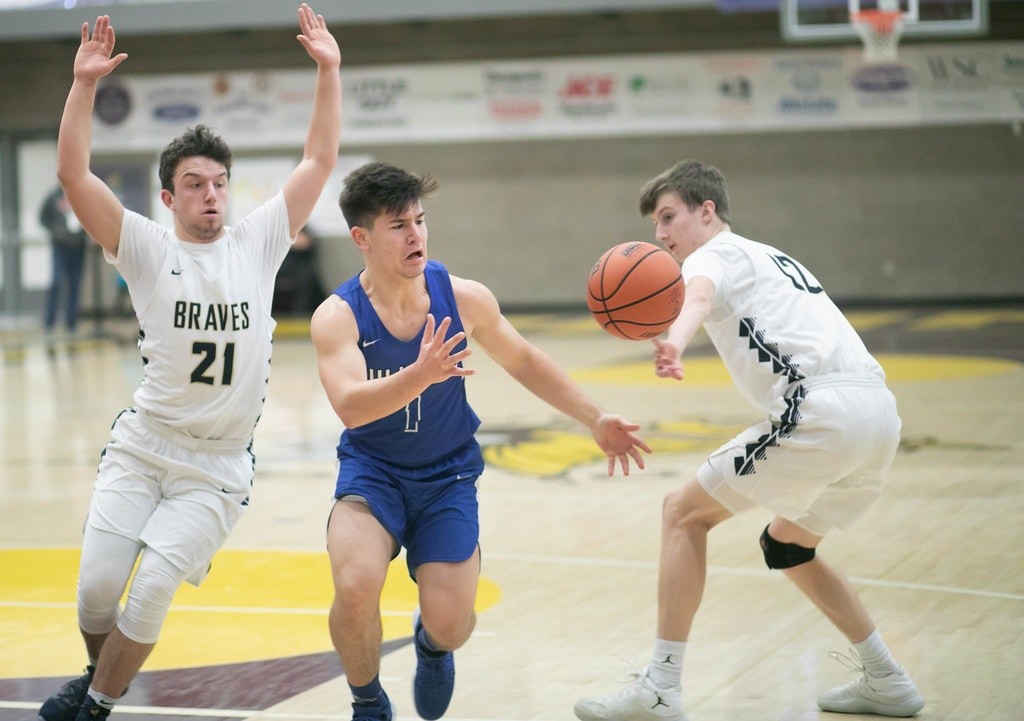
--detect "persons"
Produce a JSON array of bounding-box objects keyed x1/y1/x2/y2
[
  {"x1": 37, "y1": 183, "x2": 89, "y2": 351},
  {"x1": 31, "y1": 2, "x2": 343, "y2": 721},
  {"x1": 572, "y1": 159, "x2": 925, "y2": 721},
  {"x1": 305, "y1": 161, "x2": 657, "y2": 721}
]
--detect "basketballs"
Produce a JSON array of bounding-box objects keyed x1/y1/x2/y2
[{"x1": 585, "y1": 239, "x2": 689, "y2": 342}]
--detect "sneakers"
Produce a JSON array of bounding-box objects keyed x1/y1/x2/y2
[
  {"x1": 349, "y1": 689, "x2": 392, "y2": 719},
  {"x1": 40, "y1": 665, "x2": 115, "y2": 721},
  {"x1": 816, "y1": 647, "x2": 924, "y2": 718},
  {"x1": 412, "y1": 609, "x2": 455, "y2": 720},
  {"x1": 575, "y1": 667, "x2": 687, "y2": 721}
]
